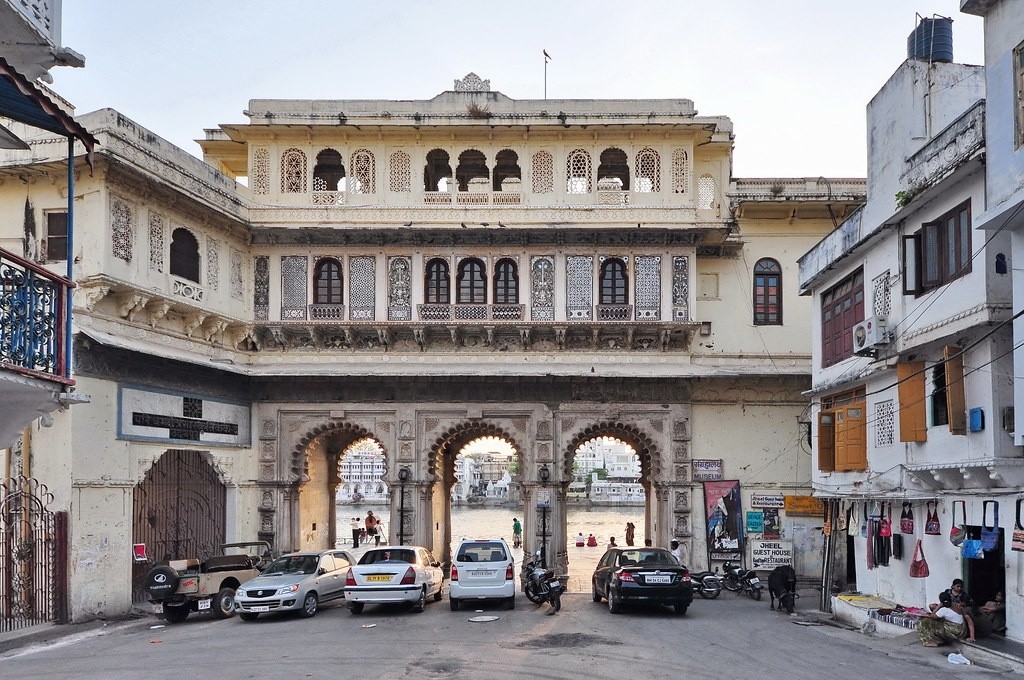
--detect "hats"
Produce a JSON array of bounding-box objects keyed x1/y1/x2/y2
[{"x1": 367, "y1": 511, "x2": 373, "y2": 515}]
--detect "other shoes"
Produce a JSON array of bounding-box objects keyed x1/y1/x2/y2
[{"x1": 921, "y1": 641, "x2": 938, "y2": 648}]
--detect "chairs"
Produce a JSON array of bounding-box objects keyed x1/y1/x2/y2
[
  {"x1": 466, "y1": 553, "x2": 478, "y2": 562},
  {"x1": 621, "y1": 555, "x2": 668, "y2": 566},
  {"x1": 491, "y1": 551, "x2": 502, "y2": 561}
]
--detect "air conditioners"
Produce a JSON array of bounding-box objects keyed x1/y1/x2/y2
[{"x1": 852, "y1": 315, "x2": 894, "y2": 359}]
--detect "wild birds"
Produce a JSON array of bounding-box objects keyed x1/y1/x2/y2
[
  {"x1": 478, "y1": 222, "x2": 489, "y2": 228},
  {"x1": 498, "y1": 221, "x2": 506, "y2": 228},
  {"x1": 591, "y1": 366, "x2": 595, "y2": 372},
  {"x1": 403, "y1": 221, "x2": 413, "y2": 227},
  {"x1": 499, "y1": 345, "x2": 508, "y2": 351},
  {"x1": 461, "y1": 222, "x2": 467, "y2": 229}
]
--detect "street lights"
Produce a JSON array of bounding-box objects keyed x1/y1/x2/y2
[
  {"x1": 540, "y1": 464, "x2": 550, "y2": 570},
  {"x1": 398, "y1": 468, "x2": 409, "y2": 546}
]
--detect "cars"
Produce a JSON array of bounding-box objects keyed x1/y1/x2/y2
[
  {"x1": 345, "y1": 545, "x2": 446, "y2": 615},
  {"x1": 450, "y1": 537, "x2": 515, "y2": 611},
  {"x1": 592, "y1": 546, "x2": 694, "y2": 616},
  {"x1": 234, "y1": 549, "x2": 357, "y2": 621}
]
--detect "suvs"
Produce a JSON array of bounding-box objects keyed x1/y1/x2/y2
[{"x1": 146, "y1": 542, "x2": 279, "y2": 624}]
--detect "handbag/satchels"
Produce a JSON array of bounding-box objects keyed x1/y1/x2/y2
[
  {"x1": 900, "y1": 502, "x2": 913, "y2": 534},
  {"x1": 879, "y1": 500, "x2": 892, "y2": 537},
  {"x1": 961, "y1": 537, "x2": 984, "y2": 559},
  {"x1": 950, "y1": 500, "x2": 966, "y2": 545},
  {"x1": 848, "y1": 500, "x2": 859, "y2": 536},
  {"x1": 837, "y1": 500, "x2": 847, "y2": 531},
  {"x1": 861, "y1": 502, "x2": 868, "y2": 537},
  {"x1": 910, "y1": 539, "x2": 929, "y2": 577},
  {"x1": 925, "y1": 501, "x2": 941, "y2": 535},
  {"x1": 981, "y1": 500, "x2": 999, "y2": 552},
  {"x1": 1011, "y1": 498, "x2": 1024, "y2": 552}
]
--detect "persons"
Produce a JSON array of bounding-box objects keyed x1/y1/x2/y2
[
  {"x1": 587, "y1": 534, "x2": 597, "y2": 546},
  {"x1": 608, "y1": 536, "x2": 617, "y2": 550},
  {"x1": 917, "y1": 578, "x2": 1004, "y2": 647},
  {"x1": 671, "y1": 541, "x2": 681, "y2": 564},
  {"x1": 512, "y1": 519, "x2": 522, "y2": 548},
  {"x1": 625, "y1": 522, "x2": 635, "y2": 546},
  {"x1": 577, "y1": 533, "x2": 584, "y2": 541},
  {"x1": 639, "y1": 539, "x2": 655, "y2": 560},
  {"x1": 350, "y1": 511, "x2": 382, "y2": 548}
]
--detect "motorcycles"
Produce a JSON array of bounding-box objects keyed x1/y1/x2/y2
[
  {"x1": 689, "y1": 566, "x2": 724, "y2": 599},
  {"x1": 721, "y1": 553, "x2": 764, "y2": 601},
  {"x1": 521, "y1": 551, "x2": 567, "y2": 612}
]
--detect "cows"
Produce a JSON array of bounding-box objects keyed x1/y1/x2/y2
[{"x1": 767, "y1": 565, "x2": 800, "y2": 614}]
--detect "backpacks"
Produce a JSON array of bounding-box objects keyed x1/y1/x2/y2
[{"x1": 374, "y1": 525, "x2": 380, "y2": 534}]
